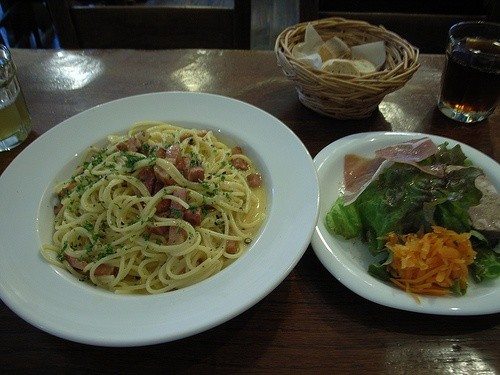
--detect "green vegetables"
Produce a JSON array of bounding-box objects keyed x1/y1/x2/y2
[{"x1": 324, "y1": 141, "x2": 500, "y2": 283}]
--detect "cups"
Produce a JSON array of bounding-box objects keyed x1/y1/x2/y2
[
  {"x1": 0, "y1": 41, "x2": 34, "y2": 156},
  {"x1": 437, "y1": 18, "x2": 499, "y2": 124}
]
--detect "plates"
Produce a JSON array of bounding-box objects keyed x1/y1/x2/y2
[
  {"x1": 310, "y1": 129, "x2": 500, "y2": 315},
  {"x1": 0, "y1": 89, "x2": 321, "y2": 350}
]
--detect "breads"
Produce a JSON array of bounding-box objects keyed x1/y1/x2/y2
[{"x1": 319, "y1": 37, "x2": 377, "y2": 75}]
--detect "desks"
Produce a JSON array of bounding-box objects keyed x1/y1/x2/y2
[{"x1": 0, "y1": 47, "x2": 499, "y2": 374}]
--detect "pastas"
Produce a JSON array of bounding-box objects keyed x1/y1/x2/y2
[{"x1": 39, "y1": 121, "x2": 266, "y2": 293}]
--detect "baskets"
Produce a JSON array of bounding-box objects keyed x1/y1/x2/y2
[{"x1": 275, "y1": 17, "x2": 421, "y2": 117}]
